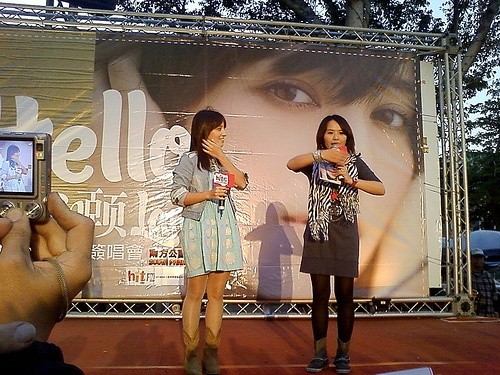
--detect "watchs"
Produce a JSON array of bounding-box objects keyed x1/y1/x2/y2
[{"x1": 350, "y1": 177, "x2": 359, "y2": 186}]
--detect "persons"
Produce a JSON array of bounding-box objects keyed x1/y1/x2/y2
[
  {"x1": 286, "y1": 114, "x2": 386, "y2": 373},
  {"x1": 95, "y1": 33, "x2": 430, "y2": 300},
  {"x1": 0, "y1": 192, "x2": 95, "y2": 375},
  {"x1": 463, "y1": 248, "x2": 500, "y2": 318},
  {"x1": 169, "y1": 105, "x2": 249, "y2": 375},
  {"x1": 1, "y1": 145, "x2": 29, "y2": 192}
]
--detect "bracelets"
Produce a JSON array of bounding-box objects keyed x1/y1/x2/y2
[{"x1": 312, "y1": 150, "x2": 323, "y2": 164}]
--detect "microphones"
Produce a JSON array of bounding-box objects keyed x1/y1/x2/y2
[
  {"x1": 218, "y1": 166, "x2": 228, "y2": 217},
  {"x1": 331, "y1": 143, "x2": 344, "y2": 179}
]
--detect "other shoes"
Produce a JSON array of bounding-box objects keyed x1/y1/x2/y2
[
  {"x1": 185, "y1": 349, "x2": 200, "y2": 375},
  {"x1": 203, "y1": 348, "x2": 219, "y2": 375}
]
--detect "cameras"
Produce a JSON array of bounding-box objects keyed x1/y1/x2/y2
[{"x1": 0, "y1": 130, "x2": 52, "y2": 223}]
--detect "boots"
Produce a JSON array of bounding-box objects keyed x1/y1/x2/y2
[
  {"x1": 334, "y1": 340, "x2": 351, "y2": 372},
  {"x1": 306, "y1": 338, "x2": 329, "y2": 372}
]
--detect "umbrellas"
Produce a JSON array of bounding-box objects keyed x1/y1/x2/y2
[{"x1": 442, "y1": 228, "x2": 500, "y2": 259}]
